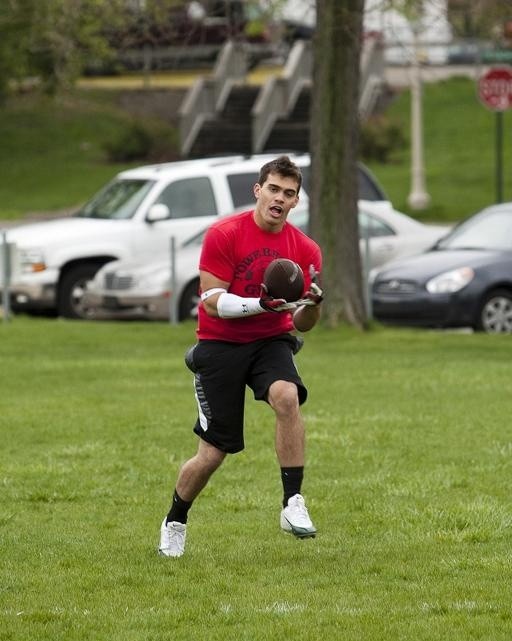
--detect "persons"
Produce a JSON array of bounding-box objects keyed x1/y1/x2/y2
[{"x1": 158, "y1": 156, "x2": 326, "y2": 558}]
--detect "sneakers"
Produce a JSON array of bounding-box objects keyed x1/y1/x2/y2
[
  {"x1": 280, "y1": 494, "x2": 316, "y2": 539},
  {"x1": 159, "y1": 514, "x2": 187, "y2": 557}
]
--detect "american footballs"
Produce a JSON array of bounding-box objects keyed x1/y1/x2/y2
[{"x1": 264, "y1": 258, "x2": 304, "y2": 302}]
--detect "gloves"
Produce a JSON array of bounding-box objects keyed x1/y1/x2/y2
[
  {"x1": 256, "y1": 283, "x2": 302, "y2": 312},
  {"x1": 302, "y1": 264, "x2": 324, "y2": 305}
]
